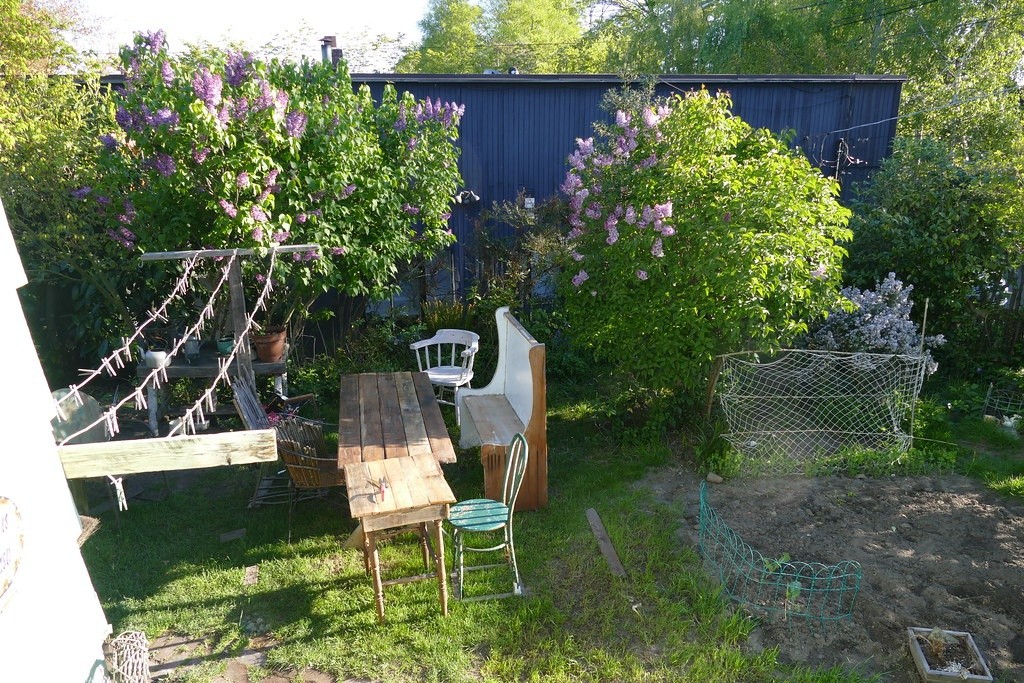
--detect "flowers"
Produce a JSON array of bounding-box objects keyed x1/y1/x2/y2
[{"x1": 70, "y1": 27, "x2": 464, "y2": 311}]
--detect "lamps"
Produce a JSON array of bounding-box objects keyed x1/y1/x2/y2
[
  {"x1": 470, "y1": 190, "x2": 480, "y2": 201},
  {"x1": 455, "y1": 192, "x2": 464, "y2": 204}
]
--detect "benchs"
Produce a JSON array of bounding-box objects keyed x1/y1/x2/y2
[{"x1": 458, "y1": 306, "x2": 548, "y2": 512}]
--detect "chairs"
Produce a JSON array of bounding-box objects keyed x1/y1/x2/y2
[
  {"x1": 410, "y1": 329, "x2": 480, "y2": 406},
  {"x1": 256, "y1": 413, "x2": 348, "y2": 545},
  {"x1": 447, "y1": 433, "x2": 529, "y2": 602}
]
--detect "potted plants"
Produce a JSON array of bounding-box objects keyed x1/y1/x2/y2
[{"x1": 907, "y1": 627, "x2": 993, "y2": 683}]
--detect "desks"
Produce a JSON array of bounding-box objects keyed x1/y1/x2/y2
[
  {"x1": 137, "y1": 347, "x2": 288, "y2": 436},
  {"x1": 336, "y1": 372, "x2": 456, "y2": 626}
]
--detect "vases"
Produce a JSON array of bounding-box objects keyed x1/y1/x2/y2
[
  {"x1": 250, "y1": 324, "x2": 287, "y2": 362},
  {"x1": 216, "y1": 337, "x2": 235, "y2": 355},
  {"x1": 185, "y1": 337, "x2": 200, "y2": 359}
]
